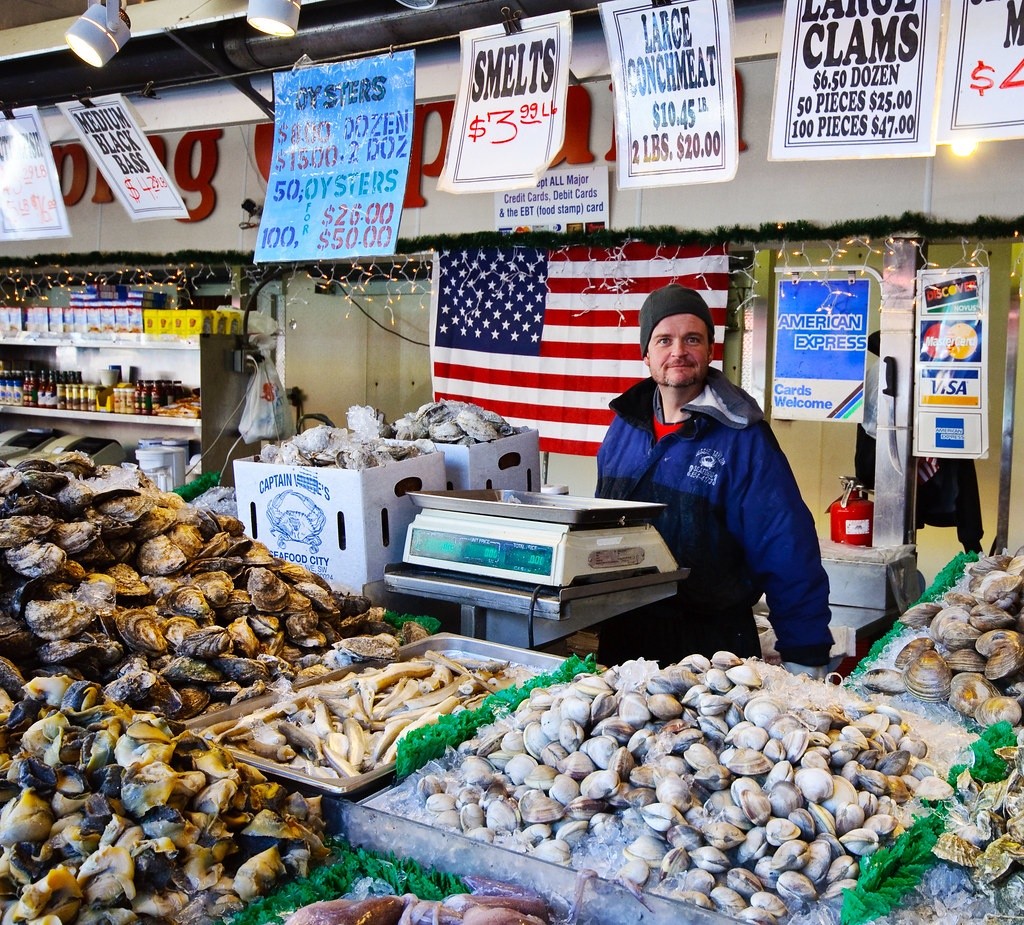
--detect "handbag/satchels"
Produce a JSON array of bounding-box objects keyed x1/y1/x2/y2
[{"x1": 238, "y1": 349, "x2": 295, "y2": 444}]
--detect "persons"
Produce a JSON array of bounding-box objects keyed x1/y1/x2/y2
[{"x1": 591, "y1": 284, "x2": 834, "y2": 686}]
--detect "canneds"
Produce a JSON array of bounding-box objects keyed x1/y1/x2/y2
[
  {"x1": 57, "y1": 384, "x2": 100, "y2": 410},
  {"x1": 113, "y1": 388, "x2": 135, "y2": 414}
]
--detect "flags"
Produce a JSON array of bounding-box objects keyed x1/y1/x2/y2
[{"x1": 426, "y1": 247, "x2": 731, "y2": 458}]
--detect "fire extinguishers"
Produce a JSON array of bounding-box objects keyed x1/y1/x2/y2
[{"x1": 830, "y1": 475, "x2": 874, "y2": 548}]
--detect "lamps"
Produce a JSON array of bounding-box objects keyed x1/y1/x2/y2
[
  {"x1": 64, "y1": 0, "x2": 134, "y2": 69},
  {"x1": 247, "y1": 0, "x2": 301, "y2": 36},
  {"x1": 396, "y1": 0, "x2": 438, "y2": 10}
]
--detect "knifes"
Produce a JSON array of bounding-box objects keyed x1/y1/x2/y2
[{"x1": 882, "y1": 356, "x2": 903, "y2": 477}]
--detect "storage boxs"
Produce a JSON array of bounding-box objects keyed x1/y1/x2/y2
[
  {"x1": 233, "y1": 437, "x2": 447, "y2": 596},
  {"x1": 820, "y1": 554, "x2": 918, "y2": 611},
  {"x1": 0, "y1": 285, "x2": 244, "y2": 335},
  {"x1": 432, "y1": 429, "x2": 541, "y2": 494}
]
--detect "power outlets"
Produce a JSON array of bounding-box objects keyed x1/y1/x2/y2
[{"x1": 233, "y1": 349, "x2": 261, "y2": 373}]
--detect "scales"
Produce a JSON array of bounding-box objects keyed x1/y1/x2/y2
[{"x1": 399, "y1": 487, "x2": 679, "y2": 598}]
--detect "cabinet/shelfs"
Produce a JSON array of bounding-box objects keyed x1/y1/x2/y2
[{"x1": 1, "y1": 333, "x2": 262, "y2": 489}]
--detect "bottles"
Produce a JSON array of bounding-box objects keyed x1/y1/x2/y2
[
  {"x1": 0, "y1": 370, "x2": 23, "y2": 406},
  {"x1": 134, "y1": 379, "x2": 184, "y2": 416},
  {"x1": 23, "y1": 370, "x2": 97, "y2": 411},
  {"x1": 113, "y1": 387, "x2": 135, "y2": 414}
]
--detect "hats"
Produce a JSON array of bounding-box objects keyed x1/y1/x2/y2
[{"x1": 640, "y1": 283, "x2": 715, "y2": 358}]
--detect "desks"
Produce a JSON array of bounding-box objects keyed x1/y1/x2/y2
[{"x1": 381, "y1": 568, "x2": 693, "y2": 654}]
